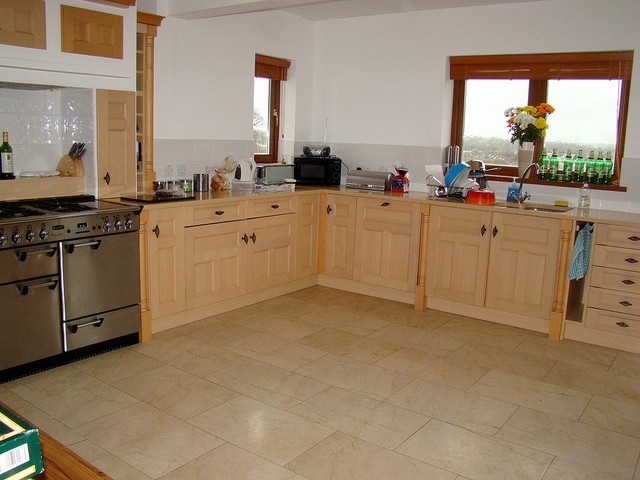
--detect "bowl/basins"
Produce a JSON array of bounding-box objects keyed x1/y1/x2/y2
[
  {"x1": 464, "y1": 190, "x2": 496, "y2": 206},
  {"x1": 444, "y1": 162, "x2": 480, "y2": 198}
]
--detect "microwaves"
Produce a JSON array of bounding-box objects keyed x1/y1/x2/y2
[{"x1": 294, "y1": 156, "x2": 341, "y2": 186}]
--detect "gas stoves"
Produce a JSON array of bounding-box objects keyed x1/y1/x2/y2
[{"x1": 0, "y1": 195, "x2": 144, "y2": 224}]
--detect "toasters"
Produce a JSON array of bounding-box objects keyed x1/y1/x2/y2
[
  {"x1": 255, "y1": 164, "x2": 294, "y2": 186},
  {"x1": 346, "y1": 169, "x2": 395, "y2": 191}
]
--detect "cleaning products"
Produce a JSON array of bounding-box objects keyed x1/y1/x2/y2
[{"x1": 506, "y1": 177, "x2": 520, "y2": 202}]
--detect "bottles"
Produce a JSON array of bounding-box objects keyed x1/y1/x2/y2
[
  {"x1": 0, "y1": 132, "x2": 13, "y2": 180},
  {"x1": 538, "y1": 147, "x2": 549, "y2": 180},
  {"x1": 193, "y1": 174, "x2": 200, "y2": 192},
  {"x1": 595, "y1": 150, "x2": 605, "y2": 184},
  {"x1": 549, "y1": 147, "x2": 558, "y2": 181},
  {"x1": 202, "y1": 174, "x2": 209, "y2": 192},
  {"x1": 562, "y1": 148, "x2": 573, "y2": 182},
  {"x1": 604, "y1": 151, "x2": 613, "y2": 184},
  {"x1": 574, "y1": 149, "x2": 584, "y2": 182},
  {"x1": 586, "y1": 151, "x2": 596, "y2": 184}
]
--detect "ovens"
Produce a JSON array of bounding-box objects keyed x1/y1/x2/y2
[{"x1": 1, "y1": 212, "x2": 140, "y2": 372}]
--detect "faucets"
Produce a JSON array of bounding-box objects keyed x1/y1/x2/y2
[{"x1": 519, "y1": 162, "x2": 540, "y2": 203}]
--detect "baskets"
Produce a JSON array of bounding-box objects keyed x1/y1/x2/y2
[{"x1": 426, "y1": 183, "x2": 470, "y2": 202}]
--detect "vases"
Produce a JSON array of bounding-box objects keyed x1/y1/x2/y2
[{"x1": 518, "y1": 141, "x2": 536, "y2": 179}]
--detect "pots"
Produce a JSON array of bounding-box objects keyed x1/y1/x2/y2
[{"x1": 465, "y1": 160, "x2": 498, "y2": 175}]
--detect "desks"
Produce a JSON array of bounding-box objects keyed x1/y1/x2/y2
[{"x1": 1, "y1": 400, "x2": 114, "y2": 480}]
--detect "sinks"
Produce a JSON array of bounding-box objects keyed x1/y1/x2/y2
[{"x1": 495, "y1": 205, "x2": 573, "y2": 213}]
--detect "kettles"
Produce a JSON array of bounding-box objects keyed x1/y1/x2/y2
[{"x1": 234, "y1": 158, "x2": 256, "y2": 181}]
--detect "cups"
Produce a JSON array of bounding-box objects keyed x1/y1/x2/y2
[{"x1": 426, "y1": 175, "x2": 444, "y2": 190}]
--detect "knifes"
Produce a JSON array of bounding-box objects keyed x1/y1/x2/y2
[{"x1": 68, "y1": 143, "x2": 87, "y2": 160}]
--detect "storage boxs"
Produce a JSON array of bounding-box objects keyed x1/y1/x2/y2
[{"x1": 0, "y1": 400, "x2": 48, "y2": 476}]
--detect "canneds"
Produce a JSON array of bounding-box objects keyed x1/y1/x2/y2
[
  {"x1": 153, "y1": 181, "x2": 166, "y2": 191},
  {"x1": 180, "y1": 179, "x2": 192, "y2": 192},
  {"x1": 168, "y1": 181, "x2": 181, "y2": 190}
]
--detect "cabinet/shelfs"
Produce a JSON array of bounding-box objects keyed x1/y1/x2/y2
[
  {"x1": 357, "y1": 194, "x2": 424, "y2": 306},
  {"x1": 424, "y1": 200, "x2": 560, "y2": 339},
  {"x1": 146, "y1": 201, "x2": 185, "y2": 335},
  {"x1": 61, "y1": 88, "x2": 137, "y2": 200},
  {"x1": 137, "y1": 9, "x2": 166, "y2": 196},
  {"x1": 297, "y1": 189, "x2": 319, "y2": 290},
  {"x1": 319, "y1": 189, "x2": 356, "y2": 294},
  {"x1": 0, "y1": 0, "x2": 137, "y2": 91},
  {"x1": 0, "y1": 174, "x2": 85, "y2": 201},
  {"x1": 560, "y1": 214, "x2": 640, "y2": 355},
  {"x1": 185, "y1": 192, "x2": 297, "y2": 324}
]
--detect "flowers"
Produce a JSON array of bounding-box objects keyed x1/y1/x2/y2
[{"x1": 504, "y1": 103, "x2": 556, "y2": 147}]
